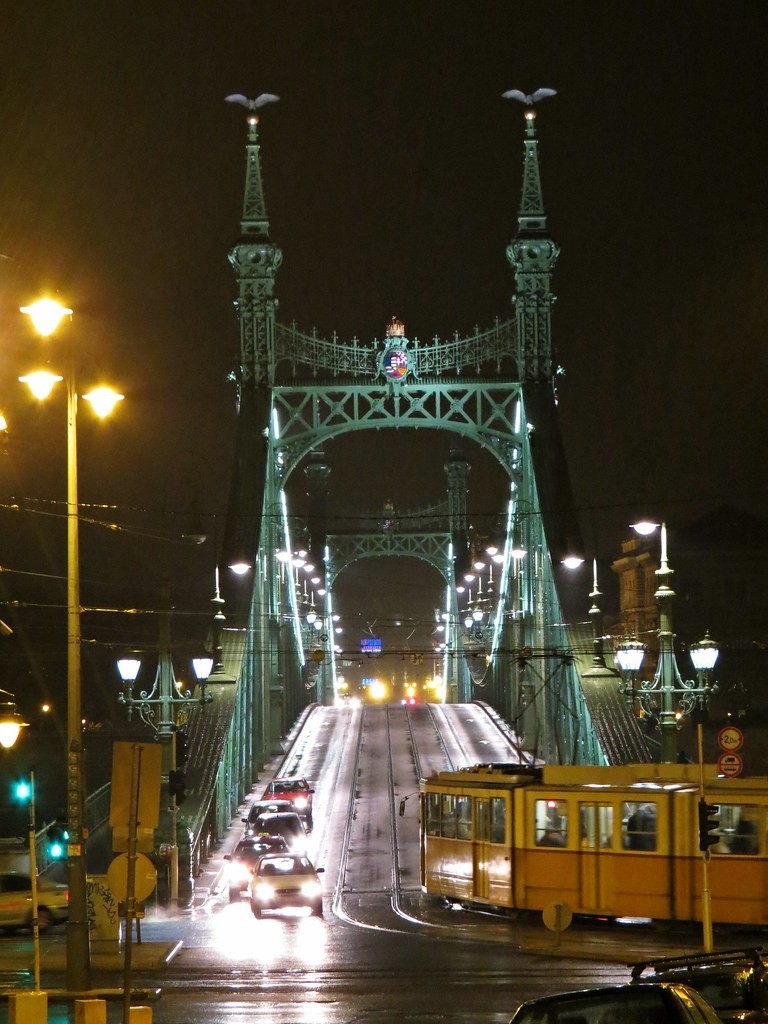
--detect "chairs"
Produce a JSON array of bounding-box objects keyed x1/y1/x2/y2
[
  {"x1": 275, "y1": 785, "x2": 284, "y2": 792},
  {"x1": 264, "y1": 864, "x2": 276, "y2": 874}
]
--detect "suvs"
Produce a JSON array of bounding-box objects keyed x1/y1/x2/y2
[{"x1": 628, "y1": 943, "x2": 768, "y2": 1024}]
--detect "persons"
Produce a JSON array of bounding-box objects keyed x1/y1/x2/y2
[
  {"x1": 428, "y1": 798, "x2": 759, "y2": 855},
  {"x1": 287, "y1": 858, "x2": 307, "y2": 875},
  {"x1": 289, "y1": 782, "x2": 302, "y2": 792}
]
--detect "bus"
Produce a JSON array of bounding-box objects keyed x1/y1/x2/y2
[{"x1": 397, "y1": 653, "x2": 768, "y2": 932}]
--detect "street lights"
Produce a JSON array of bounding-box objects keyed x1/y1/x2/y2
[
  {"x1": 200, "y1": 514, "x2": 255, "y2": 683},
  {"x1": 553, "y1": 544, "x2": 621, "y2": 678},
  {"x1": 615, "y1": 514, "x2": 719, "y2": 763},
  {"x1": 114, "y1": 582, "x2": 216, "y2": 899},
  {"x1": 18, "y1": 355, "x2": 126, "y2": 1014}
]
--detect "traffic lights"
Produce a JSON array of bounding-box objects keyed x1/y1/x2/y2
[
  {"x1": 696, "y1": 797, "x2": 723, "y2": 852},
  {"x1": 174, "y1": 730, "x2": 190, "y2": 768},
  {"x1": 167, "y1": 769, "x2": 188, "y2": 807}
]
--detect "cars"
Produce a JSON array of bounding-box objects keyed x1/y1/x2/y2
[
  {"x1": 0, "y1": 868, "x2": 69, "y2": 934},
  {"x1": 240, "y1": 799, "x2": 311, "y2": 856},
  {"x1": 508, "y1": 982, "x2": 727, "y2": 1024},
  {"x1": 223, "y1": 832, "x2": 296, "y2": 897},
  {"x1": 242, "y1": 852, "x2": 326, "y2": 919},
  {"x1": 263, "y1": 775, "x2": 316, "y2": 827}
]
